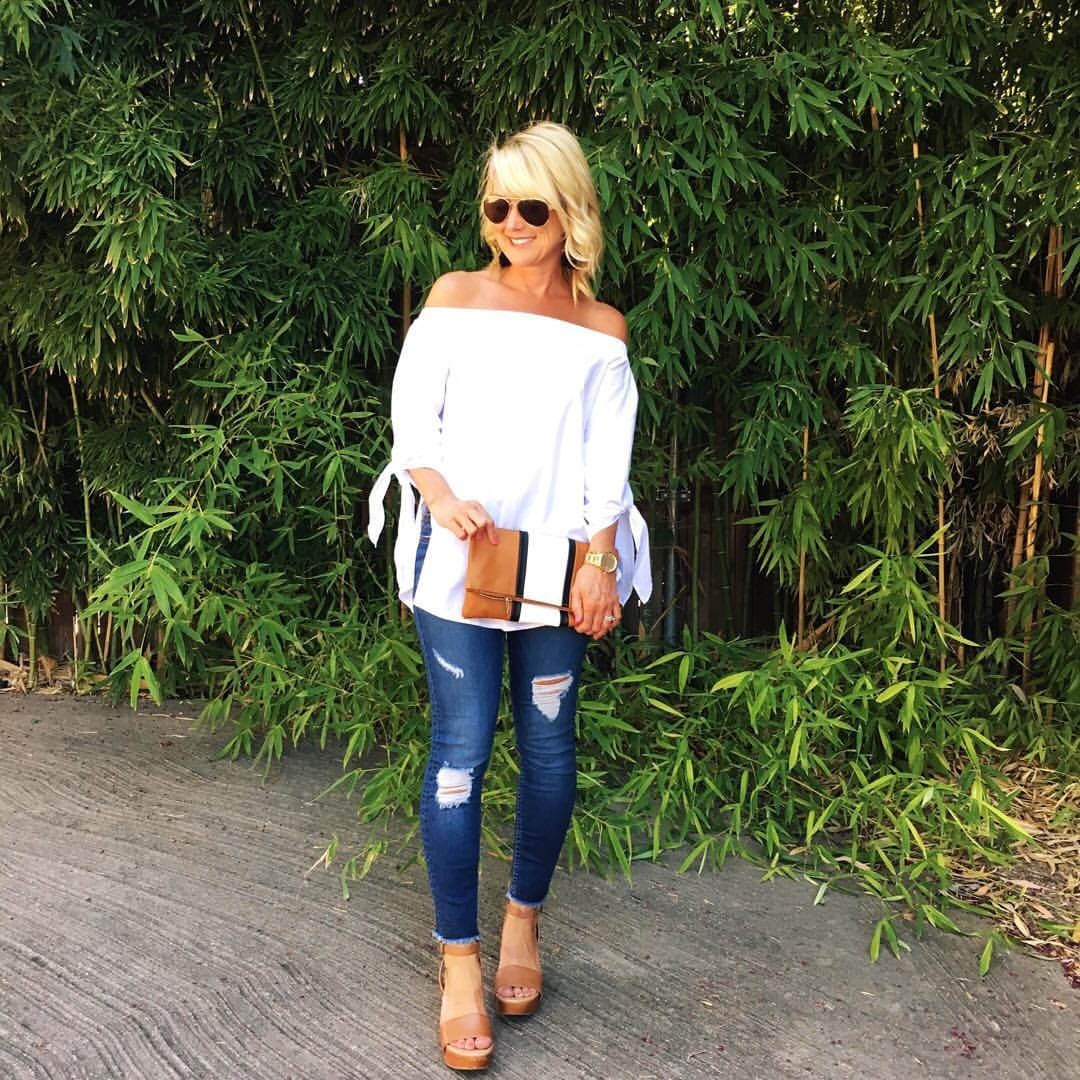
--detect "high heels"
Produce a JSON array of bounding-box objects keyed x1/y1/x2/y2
[
  {"x1": 436, "y1": 941, "x2": 494, "y2": 1071},
  {"x1": 491, "y1": 904, "x2": 543, "y2": 1016}
]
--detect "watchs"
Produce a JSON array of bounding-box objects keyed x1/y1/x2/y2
[{"x1": 585, "y1": 552, "x2": 618, "y2": 573}]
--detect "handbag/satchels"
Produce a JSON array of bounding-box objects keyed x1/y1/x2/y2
[{"x1": 462, "y1": 526, "x2": 620, "y2": 627}]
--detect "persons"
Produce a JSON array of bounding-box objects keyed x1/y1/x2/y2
[{"x1": 369, "y1": 125, "x2": 653, "y2": 1069}]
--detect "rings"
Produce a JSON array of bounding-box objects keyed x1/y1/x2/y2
[{"x1": 604, "y1": 615, "x2": 613, "y2": 621}]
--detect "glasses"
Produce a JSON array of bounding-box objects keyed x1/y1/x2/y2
[{"x1": 483, "y1": 198, "x2": 556, "y2": 229}]
